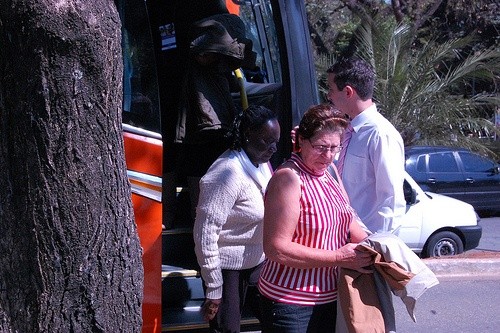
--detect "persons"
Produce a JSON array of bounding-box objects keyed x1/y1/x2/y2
[{"x1": 145, "y1": 0, "x2": 404, "y2": 333}]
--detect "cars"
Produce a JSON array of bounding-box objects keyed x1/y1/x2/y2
[{"x1": 397, "y1": 169, "x2": 483, "y2": 259}]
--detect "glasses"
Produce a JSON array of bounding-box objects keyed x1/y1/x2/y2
[{"x1": 309, "y1": 138, "x2": 344, "y2": 153}]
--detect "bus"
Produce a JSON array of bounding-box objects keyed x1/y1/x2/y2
[{"x1": 116, "y1": 0, "x2": 323, "y2": 333}]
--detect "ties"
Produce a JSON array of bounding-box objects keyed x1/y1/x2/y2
[{"x1": 337, "y1": 126, "x2": 353, "y2": 179}]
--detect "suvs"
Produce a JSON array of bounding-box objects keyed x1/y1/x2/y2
[{"x1": 405, "y1": 145, "x2": 500, "y2": 217}]
